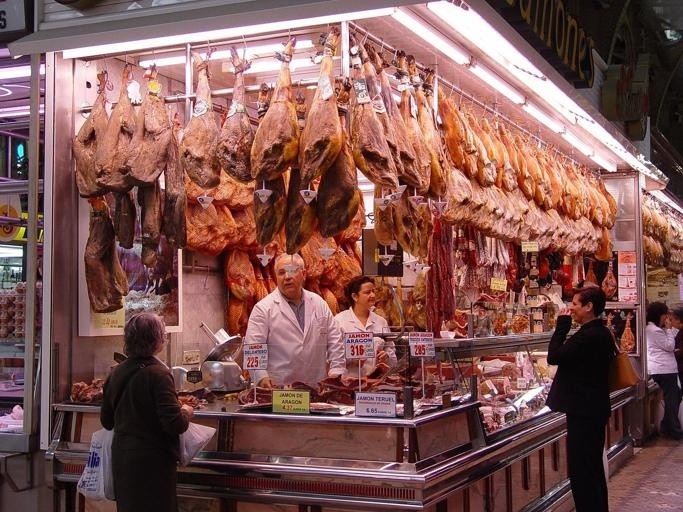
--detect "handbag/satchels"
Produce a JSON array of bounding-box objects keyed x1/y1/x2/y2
[{"x1": 610, "y1": 352, "x2": 639, "y2": 392}]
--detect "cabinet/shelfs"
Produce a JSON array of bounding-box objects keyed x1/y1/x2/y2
[{"x1": 407, "y1": 327, "x2": 615, "y2": 447}]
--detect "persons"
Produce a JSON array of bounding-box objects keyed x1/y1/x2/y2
[
  {"x1": 668, "y1": 309, "x2": 683, "y2": 389},
  {"x1": 643, "y1": 300, "x2": 683, "y2": 439},
  {"x1": 329, "y1": 274, "x2": 398, "y2": 384},
  {"x1": 243, "y1": 252, "x2": 346, "y2": 392},
  {"x1": 99, "y1": 311, "x2": 195, "y2": 511},
  {"x1": 545, "y1": 282, "x2": 617, "y2": 512}
]
